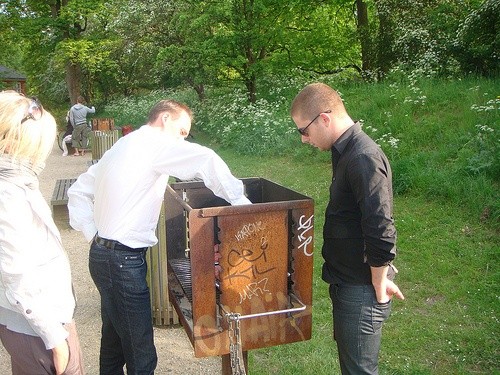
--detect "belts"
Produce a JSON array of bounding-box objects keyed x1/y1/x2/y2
[{"x1": 96, "y1": 236, "x2": 148, "y2": 253}]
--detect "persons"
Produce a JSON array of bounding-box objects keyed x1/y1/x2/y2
[
  {"x1": 61, "y1": 111, "x2": 77, "y2": 157},
  {"x1": 292, "y1": 82, "x2": 405, "y2": 375},
  {"x1": 68, "y1": 99, "x2": 252, "y2": 374},
  {"x1": 69, "y1": 95, "x2": 95, "y2": 156},
  {"x1": 0, "y1": 90, "x2": 84, "y2": 374}
]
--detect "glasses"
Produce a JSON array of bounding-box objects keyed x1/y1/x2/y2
[
  {"x1": 2, "y1": 96, "x2": 43, "y2": 140},
  {"x1": 298, "y1": 110, "x2": 332, "y2": 137}
]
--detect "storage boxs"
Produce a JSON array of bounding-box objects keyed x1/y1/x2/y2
[
  {"x1": 162, "y1": 177, "x2": 315, "y2": 359},
  {"x1": 91, "y1": 117, "x2": 115, "y2": 131}
]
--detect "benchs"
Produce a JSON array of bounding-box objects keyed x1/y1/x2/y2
[{"x1": 51, "y1": 158, "x2": 101, "y2": 224}]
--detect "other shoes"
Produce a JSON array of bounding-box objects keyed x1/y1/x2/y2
[
  {"x1": 81, "y1": 153, "x2": 85, "y2": 156},
  {"x1": 74, "y1": 153, "x2": 79, "y2": 156}
]
